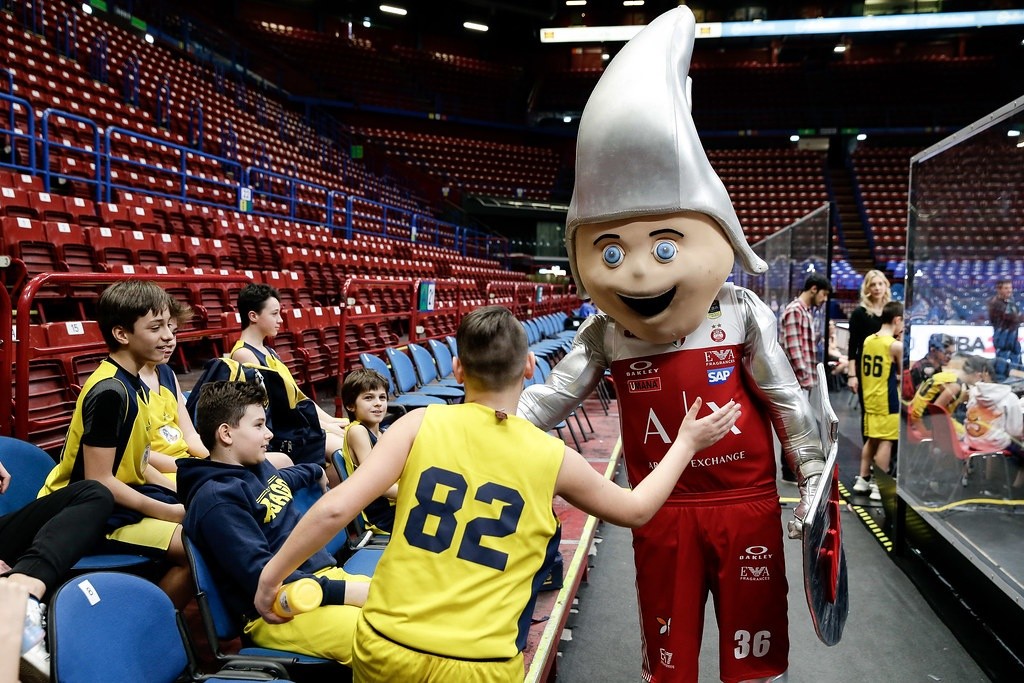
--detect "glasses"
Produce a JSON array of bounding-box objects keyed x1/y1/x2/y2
[{"x1": 936, "y1": 348, "x2": 957, "y2": 358}]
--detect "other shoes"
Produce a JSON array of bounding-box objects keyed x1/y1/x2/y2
[
  {"x1": 22, "y1": 595, "x2": 51, "y2": 680},
  {"x1": 983, "y1": 487, "x2": 1002, "y2": 498},
  {"x1": 782, "y1": 473, "x2": 799, "y2": 481},
  {"x1": 1007, "y1": 487, "x2": 1024, "y2": 499}
]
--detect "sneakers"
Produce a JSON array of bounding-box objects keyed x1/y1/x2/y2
[{"x1": 852, "y1": 475, "x2": 882, "y2": 500}]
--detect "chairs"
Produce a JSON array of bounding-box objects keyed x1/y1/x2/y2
[{"x1": 0, "y1": 0, "x2": 1024, "y2": 683}]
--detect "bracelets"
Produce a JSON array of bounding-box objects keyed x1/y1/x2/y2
[{"x1": 847, "y1": 375, "x2": 857, "y2": 378}]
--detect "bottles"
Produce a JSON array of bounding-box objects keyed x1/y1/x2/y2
[{"x1": 272, "y1": 577, "x2": 323, "y2": 619}]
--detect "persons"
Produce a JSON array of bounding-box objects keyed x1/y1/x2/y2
[
  {"x1": 847, "y1": 270, "x2": 891, "y2": 473},
  {"x1": 37, "y1": 280, "x2": 197, "y2": 614},
  {"x1": 229, "y1": 284, "x2": 350, "y2": 462},
  {"x1": 514, "y1": 5, "x2": 826, "y2": 683},
  {"x1": 138, "y1": 293, "x2": 373, "y2": 551},
  {"x1": 853, "y1": 302, "x2": 904, "y2": 500},
  {"x1": 989, "y1": 279, "x2": 1024, "y2": 381},
  {"x1": 341, "y1": 369, "x2": 401, "y2": 535},
  {"x1": 175, "y1": 381, "x2": 372, "y2": 669},
  {"x1": 911, "y1": 333, "x2": 1024, "y2": 514},
  {"x1": 579, "y1": 298, "x2": 607, "y2": 317},
  {"x1": 829, "y1": 319, "x2": 848, "y2": 386},
  {"x1": 778, "y1": 274, "x2": 833, "y2": 485},
  {"x1": 254, "y1": 304, "x2": 742, "y2": 683},
  {"x1": 0, "y1": 461, "x2": 115, "y2": 683}
]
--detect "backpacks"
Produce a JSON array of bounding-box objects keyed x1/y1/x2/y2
[{"x1": 186, "y1": 359, "x2": 326, "y2": 465}]
